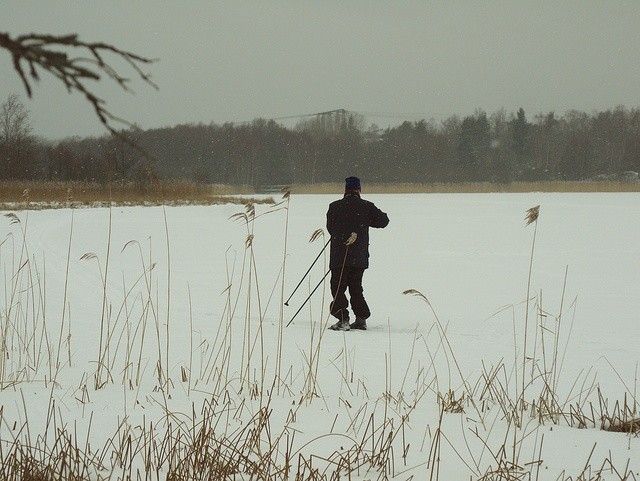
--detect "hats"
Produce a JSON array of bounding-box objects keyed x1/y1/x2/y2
[{"x1": 345, "y1": 176, "x2": 361, "y2": 191}]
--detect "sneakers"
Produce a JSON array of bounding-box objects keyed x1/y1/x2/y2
[
  {"x1": 331, "y1": 320, "x2": 350, "y2": 330},
  {"x1": 350, "y1": 318, "x2": 367, "y2": 329}
]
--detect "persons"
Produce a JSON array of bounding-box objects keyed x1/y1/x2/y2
[{"x1": 326, "y1": 178, "x2": 389, "y2": 331}]
